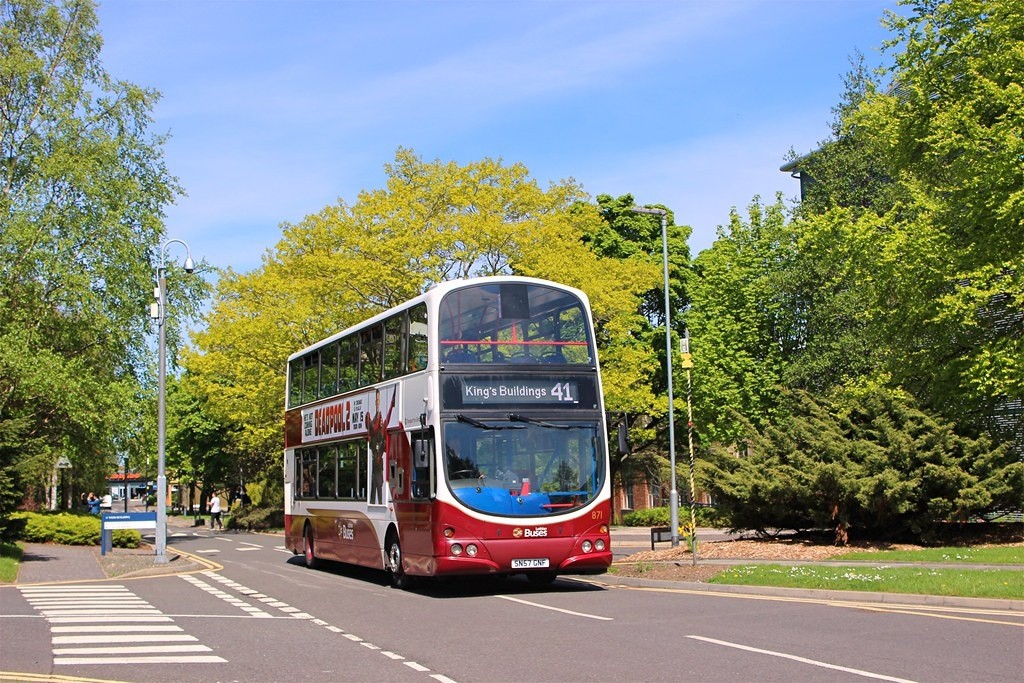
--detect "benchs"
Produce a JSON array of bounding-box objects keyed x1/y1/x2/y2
[{"x1": 651, "y1": 525, "x2": 689, "y2": 551}]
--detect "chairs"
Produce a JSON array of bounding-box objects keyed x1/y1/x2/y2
[
  {"x1": 320, "y1": 352, "x2": 575, "y2": 399},
  {"x1": 299, "y1": 458, "x2": 367, "y2": 500}
]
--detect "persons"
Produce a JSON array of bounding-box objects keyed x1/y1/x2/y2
[
  {"x1": 236, "y1": 488, "x2": 251, "y2": 505},
  {"x1": 142, "y1": 494, "x2": 145, "y2": 503},
  {"x1": 447, "y1": 436, "x2": 484, "y2": 479},
  {"x1": 365, "y1": 385, "x2": 397, "y2": 504},
  {"x1": 297, "y1": 464, "x2": 328, "y2": 497},
  {"x1": 830, "y1": 505, "x2": 851, "y2": 547},
  {"x1": 81, "y1": 492, "x2": 104, "y2": 514},
  {"x1": 207, "y1": 492, "x2": 223, "y2": 530}
]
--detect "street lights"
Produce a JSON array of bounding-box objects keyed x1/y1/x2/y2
[
  {"x1": 124, "y1": 452, "x2": 130, "y2": 513},
  {"x1": 155, "y1": 240, "x2": 195, "y2": 563},
  {"x1": 621, "y1": 206, "x2": 680, "y2": 550}
]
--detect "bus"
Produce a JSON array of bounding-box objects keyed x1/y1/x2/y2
[{"x1": 284, "y1": 275, "x2": 633, "y2": 593}]
